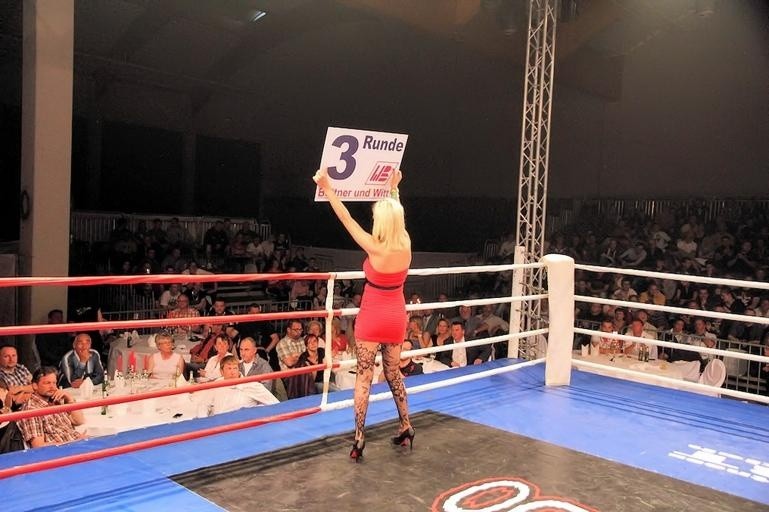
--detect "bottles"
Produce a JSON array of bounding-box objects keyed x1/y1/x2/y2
[
  {"x1": 175, "y1": 367, "x2": 180, "y2": 387},
  {"x1": 101, "y1": 370, "x2": 110, "y2": 415},
  {"x1": 638, "y1": 346, "x2": 649, "y2": 362},
  {"x1": 189, "y1": 370, "x2": 194, "y2": 393},
  {"x1": 127, "y1": 331, "x2": 132, "y2": 348}
]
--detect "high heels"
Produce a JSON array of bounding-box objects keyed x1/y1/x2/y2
[
  {"x1": 391, "y1": 427, "x2": 415, "y2": 449},
  {"x1": 350, "y1": 440, "x2": 365, "y2": 463}
]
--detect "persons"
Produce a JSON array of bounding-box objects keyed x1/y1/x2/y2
[
  {"x1": 377, "y1": 291, "x2": 513, "y2": 384},
  {"x1": 466, "y1": 205, "x2": 769, "y2": 406},
  {"x1": 312, "y1": 168, "x2": 415, "y2": 464},
  {"x1": 1, "y1": 213, "x2": 362, "y2": 457}
]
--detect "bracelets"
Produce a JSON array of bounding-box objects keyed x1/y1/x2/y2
[{"x1": 390, "y1": 188, "x2": 400, "y2": 200}]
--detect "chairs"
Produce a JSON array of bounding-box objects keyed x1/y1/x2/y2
[{"x1": 16, "y1": 291, "x2": 748, "y2": 451}]
